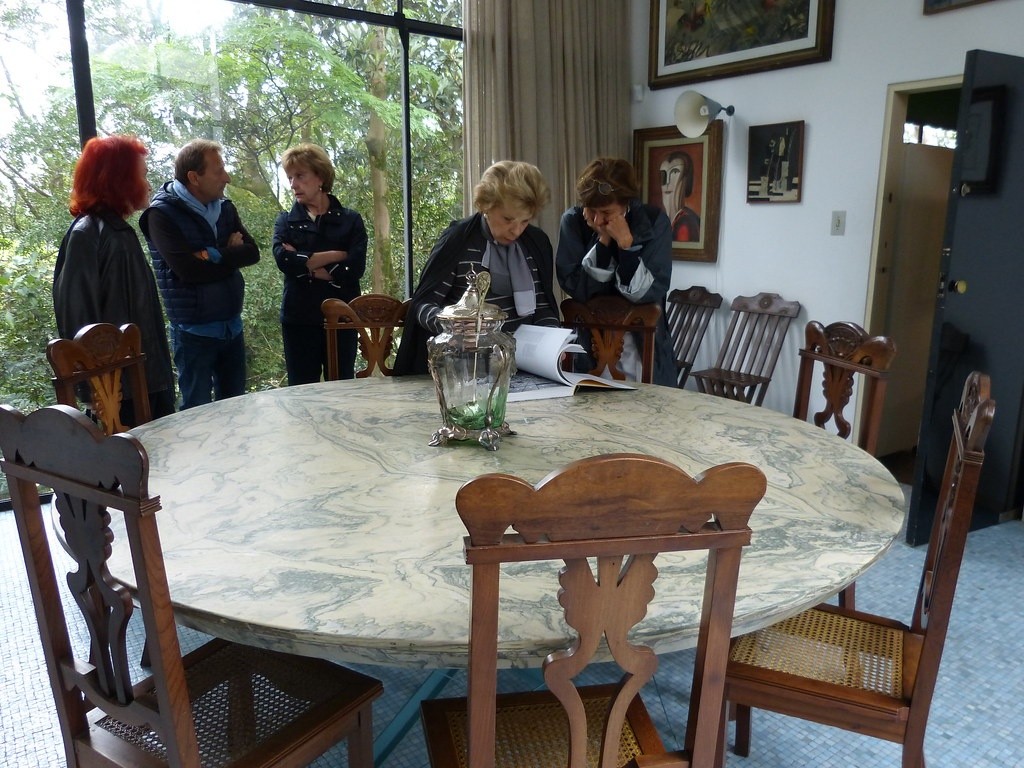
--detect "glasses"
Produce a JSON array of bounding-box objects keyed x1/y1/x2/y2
[{"x1": 582, "y1": 177, "x2": 615, "y2": 195}]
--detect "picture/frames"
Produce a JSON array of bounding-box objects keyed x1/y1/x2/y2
[
  {"x1": 746, "y1": 120, "x2": 804, "y2": 203},
  {"x1": 646, "y1": 0, "x2": 837, "y2": 91},
  {"x1": 633, "y1": 116, "x2": 724, "y2": 263}
]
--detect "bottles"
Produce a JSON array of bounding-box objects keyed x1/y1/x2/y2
[{"x1": 422, "y1": 264, "x2": 517, "y2": 451}]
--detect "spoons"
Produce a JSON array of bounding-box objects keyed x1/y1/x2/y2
[{"x1": 463, "y1": 272, "x2": 492, "y2": 417}]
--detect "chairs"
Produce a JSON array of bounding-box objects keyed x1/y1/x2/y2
[
  {"x1": 325, "y1": 294, "x2": 416, "y2": 381},
  {"x1": 685, "y1": 292, "x2": 798, "y2": 406},
  {"x1": 698, "y1": 369, "x2": 995, "y2": 767},
  {"x1": 666, "y1": 285, "x2": 723, "y2": 388},
  {"x1": 563, "y1": 299, "x2": 667, "y2": 386},
  {"x1": 0, "y1": 405, "x2": 385, "y2": 767},
  {"x1": 703, "y1": 321, "x2": 895, "y2": 718},
  {"x1": 46, "y1": 323, "x2": 149, "y2": 446},
  {"x1": 420, "y1": 455, "x2": 769, "y2": 767}
]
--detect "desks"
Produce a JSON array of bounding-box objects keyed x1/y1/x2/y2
[{"x1": 102, "y1": 383, "x2": 915, "y2": 673}]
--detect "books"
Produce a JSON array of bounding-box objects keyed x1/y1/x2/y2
[{"x1": 488, "y1": 323, "x2": 637, "y2": 402}]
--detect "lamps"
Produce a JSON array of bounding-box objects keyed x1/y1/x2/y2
[{"x1": 673, "y1": 89, "x2": 735, "y2": 138}]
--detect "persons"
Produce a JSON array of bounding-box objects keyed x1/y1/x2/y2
[
  {"x1": 271, "y1": 144, "x2": 369, "y2": 387},
  {"x1": 555, "y1": 157, "x2": 677, "y2": 390},
  {"x1": 138, "y1": 137, "x2": 260, "y2": 412},
  {"x1": 391, "y1": 161, "x2": 566, "y2": 382},
  {"x1": 51, "y1": 135, "x2": 175, "y2": 431}
]
